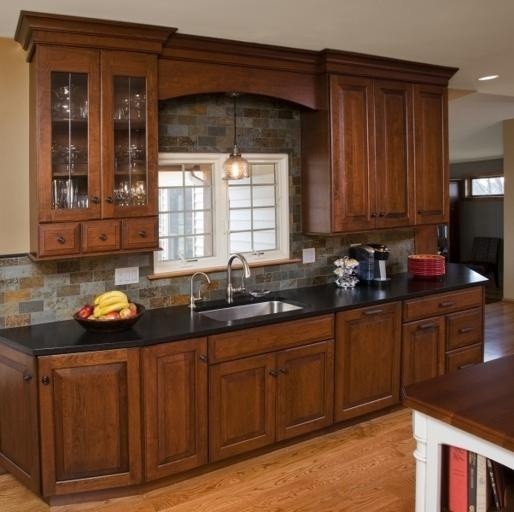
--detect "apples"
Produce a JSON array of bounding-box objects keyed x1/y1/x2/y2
[{"x1": 78, "y1": 302, "x2": 137, "y2": 321}]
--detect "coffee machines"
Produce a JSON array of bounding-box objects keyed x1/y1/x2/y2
[{"x1": 348, "y1": 241, "x2": 391, "y2": 282}]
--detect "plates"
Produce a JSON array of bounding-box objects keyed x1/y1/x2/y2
[{"x1": 406, "y1": 253, "x2": 446, "y2": 279}]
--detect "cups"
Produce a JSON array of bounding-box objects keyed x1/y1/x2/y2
[{"x1": 49, "y1": 179, "x2": 89, "y2": 210}]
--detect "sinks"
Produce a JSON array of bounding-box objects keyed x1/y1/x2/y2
[{"x1": 193, "y1": 297, "x2": 305, "y2": 322}]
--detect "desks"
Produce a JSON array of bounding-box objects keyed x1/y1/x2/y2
[{"x1": 398, "y1": 354, "x2": 514, "y2": 512}]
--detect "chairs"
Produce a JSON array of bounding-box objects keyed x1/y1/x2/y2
[{"x1": 464, "y1": 236, "x2": 501, "y2": 289}]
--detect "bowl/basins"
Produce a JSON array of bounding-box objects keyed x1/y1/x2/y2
[{"x1": 73, "y1": 302, "x2": 144, "y2": 333}]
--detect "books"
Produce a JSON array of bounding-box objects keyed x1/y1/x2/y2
[{"x1": 447, "y1": 444, "x2": 512, "y2": 511}]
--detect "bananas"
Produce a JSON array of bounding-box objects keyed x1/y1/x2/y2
[{"x1": 93, "y1": 290, "x2": 128, "y2": 318}]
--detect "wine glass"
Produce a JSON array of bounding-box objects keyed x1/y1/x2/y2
[
  {"x1": 118, "y1": 94, "x2": 144, "y2": 119},
  {"x1": 115, "y1": 180, "x2": 146, "y2": 206}
]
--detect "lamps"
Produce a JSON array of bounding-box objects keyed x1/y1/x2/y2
[{"x1": 221, "y1": 93, "x2": 249, "y2": 181}]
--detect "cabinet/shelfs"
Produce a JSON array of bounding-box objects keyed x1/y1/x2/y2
[
  {"x1": 303, "y1": 48, "x2": 460, "y2": 237},
  {"x1": 140, "y1": 336, "x2": 210, "y2": 483},
  {"x1": 211, "y1": 313, "x2": 334, "y2": 461},
  {"x1": 334, "y1": 301, "x2": 402, "y2": 424},
  {"x1": 402, "y1": 285, "x2": 486, "y2": 401},
  {"x1": 13, "y1": 10, "x2": 176, "y2": 263},
  {"x1": 1, "y1": 339, "x2": 142, "y2": 507}
]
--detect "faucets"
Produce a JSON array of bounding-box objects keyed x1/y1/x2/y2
[
  {"x1": 224, "y1": 254, "x2": 251, "y2": 304},
  {"x1": 188, "y1": 271, "x2": 211, "y2": 308}
]
